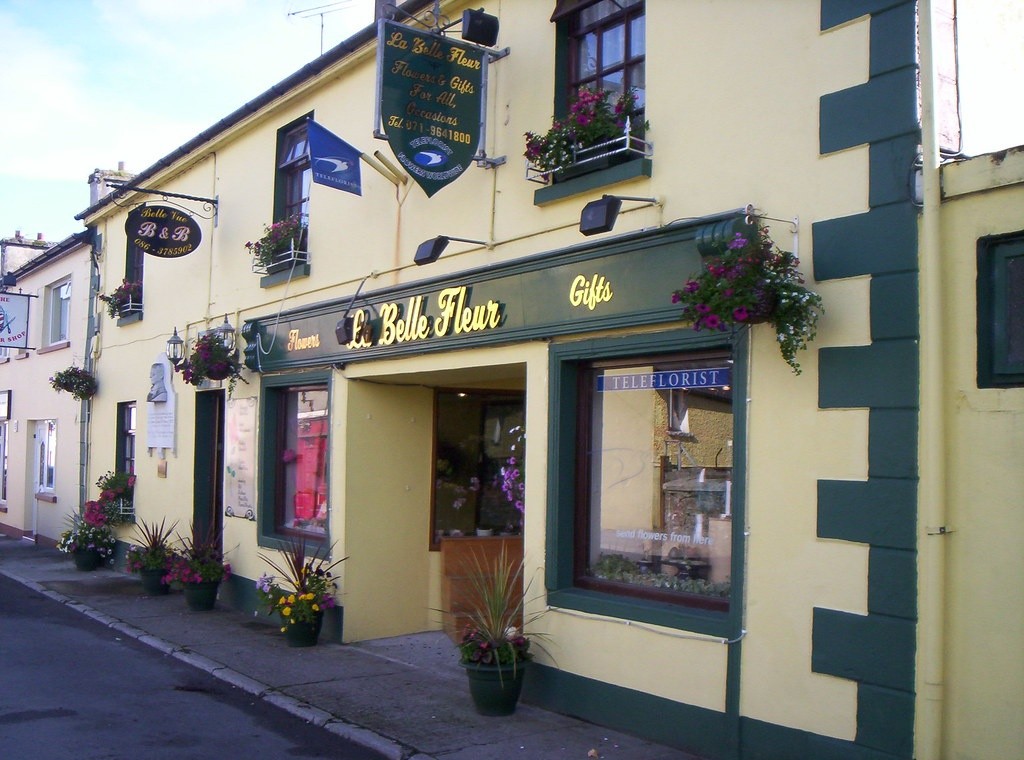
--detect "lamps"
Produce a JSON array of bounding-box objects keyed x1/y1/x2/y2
[
  {"x1": 439, "y1": 5, "x2": 499, "y2": 48},
  {"x1": 413, "y1": 235, "x2": 490, "y2": 266},
  {"x1": 166, "y1": 326, "x2": 185, "y2": 368},
  {"x1": 580, "y1": 193, "x2": 657, "y2": 237},
  {"x1": 2, "y1": 271, "x2": 17, "y2": 289},
  {"x1": 216, "y1": 313, "x2": 235, "y2": 352},
  {"x1": 335, "y1": 276, "x2": 371, "y2": 345}
]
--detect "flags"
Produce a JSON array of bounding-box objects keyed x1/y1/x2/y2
[{"x1": 307, "y1": 120, "x2": 362, "y2": 196}]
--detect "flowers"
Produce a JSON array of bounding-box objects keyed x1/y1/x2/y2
[
  {"x1": 97, "y1": 278, "x2": 142, "y2": 319},
  {"x1": 243, "y1": 211, "x2": 308, "y2": 265},
  {"x1": 435, "y1": 458, "x2": 482, "y2": 510},
  {"x1": 423, "y1": 539, "x2": 564, "y2": 689},
  {"x1": 492, "y1": 423, "x2": 525, "y2": 528},
  {"x1": 522, "y1": 82, "x2": 650, "y2": 185},
  {"x1": 174, "y1": 518, "x2": 240, "y2": 584},
  {"x1": 95, "y1": 465, "x2": 137, "y2": 529},
  {"x1": 56, "y1": 500, "x2": 117, "y2": 572},
  {"x1": 123, "y1": 514, "x2": 187, "y2": 577},
  {"x1": 671, "y1": 209, "x2": 824, "y2": 376},
  {"x1": 48, "y1": 363, "x2": 99, "y2": 402},
  {"x1": 255, "y1": 532, "x2": 351, "y2": 635},
  {"x1": 175, "y1": 332, "x2": 250, "y2": 401}
]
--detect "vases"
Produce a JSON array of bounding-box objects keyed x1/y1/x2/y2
[
  {"x1": 184, "y1": 582, "x2": 219, "y2": 612},
  {"x1": 118, "y1": 299, "x2": 142, "y2": 318},
  {"x1": 266, "y1": 248, "x2": 306, "y2": 274},
  {"x1": 279, "y1": 610, "x2": 324, "y2": 648},
  {"x1": 72, "y1": 550, "x2": 99, "y2": 572},
  {"x1": 457, "y1": 658, "x2": 533, "y2": 718},
  {"x1": 140, "y1": 569, "x2": 171, "y2": 597},
  {"x1": 553, "y1": 143, "x2": 628, "y2": 183}
]
